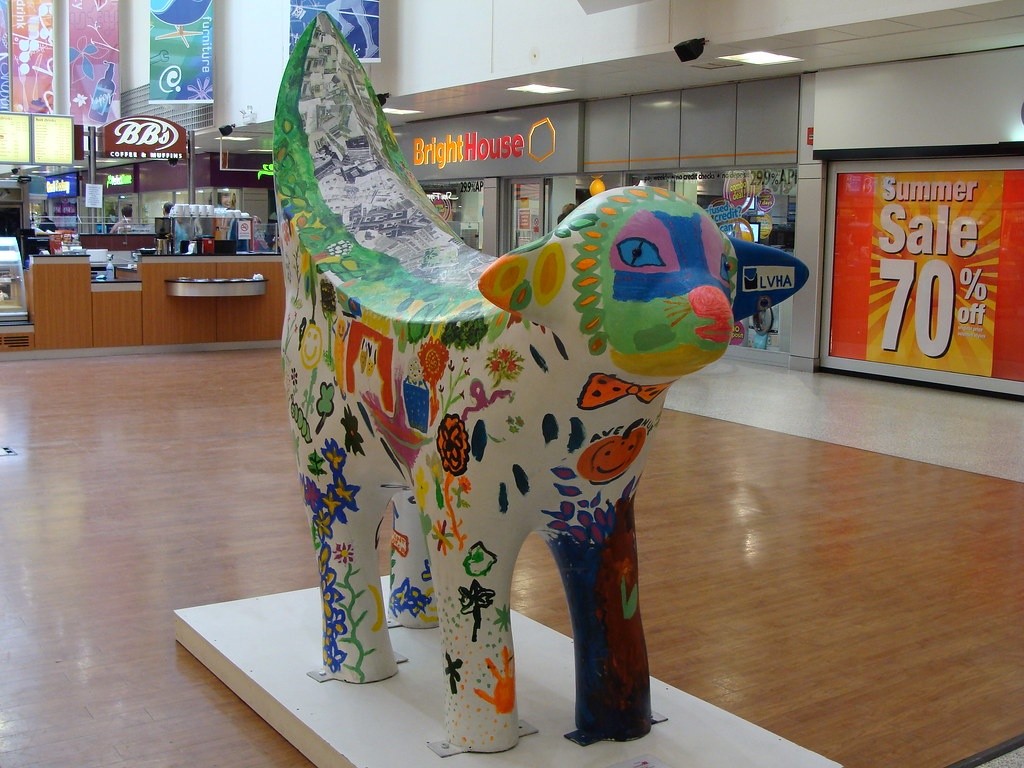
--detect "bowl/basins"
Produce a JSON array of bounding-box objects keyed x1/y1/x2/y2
[{"x1": 141, "y1": 249, "x2": 156, "y2": 255}]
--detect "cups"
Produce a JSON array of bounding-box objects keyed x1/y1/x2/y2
[{"x1": 170, "y1": 203, "x2": 250, "y2": 217}]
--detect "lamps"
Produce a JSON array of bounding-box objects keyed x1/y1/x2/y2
[
  {"x1": 377, "y1": 92, "x2": 389, "y2": 107},
  {"x1": 240, "y1": 105, "x2": 257, "y2": 125},
  {"x1": 218, "y1": 123, "x2": 236, "y2": 137},
  {"x1": 674, "y1": 37, "x2": 709, "y2": 62},
  {"x1": 11, "y1": 168, "x2": 20, "y2": 174},
  {"x1": 168, "y1": 158, "x2": 178, "y2": 167}
]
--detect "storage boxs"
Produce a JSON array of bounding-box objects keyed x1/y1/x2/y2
[
  {"x1": 87, "y1": 249, "x2": 109, "y2": 262},
  {"x1": 153, "y1": 217, "x2": 280, "y2": 255}
]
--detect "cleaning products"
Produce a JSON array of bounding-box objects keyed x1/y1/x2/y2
[{"x1": 105, "y1": 252, "x2": 115, "y2": 281}]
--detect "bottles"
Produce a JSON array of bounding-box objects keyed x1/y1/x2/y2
[{"x1": 88, "y1": 60, "x2": 116, "y2": 124}]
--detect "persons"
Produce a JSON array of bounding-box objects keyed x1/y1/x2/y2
[
  {"x1": 209, "y1": 190, "x2": 237, "y2": 210},
  {"x1": 160, "y1": 202, "x2": 175, "y2": 217},
  {"x1": 110, "y1": 204, "x2": 135, "y2": 233},
  {"x1": 557, "y1": 203, "x2": 577, "y2": 226},
  {"x1": 253, "y1": 211, "x2": 281, "y2": 252},
  {"x1": 38, "y1": 212, "x2": 58, "y2": 232}
]
